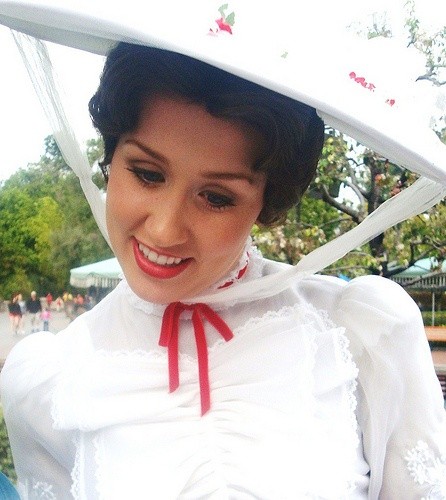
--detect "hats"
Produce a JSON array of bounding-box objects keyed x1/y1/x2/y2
[{"x1": 0, "y1": 0, "x2": 446, "y2": 185}]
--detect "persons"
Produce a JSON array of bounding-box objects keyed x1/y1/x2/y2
[
  {"x1": 6, "y1": 285, "x2": 98, "y2": 335},
  {"x1": 0, "y1": 0, "x2": 446, "y2": 500}
]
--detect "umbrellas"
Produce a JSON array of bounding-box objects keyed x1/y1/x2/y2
[
  {"x1": 394, "y1": 258, "x2": 446, "y2": 328},
  {"x1": 69, "y1": 257, "x2": 123, "y2": 288}
]
